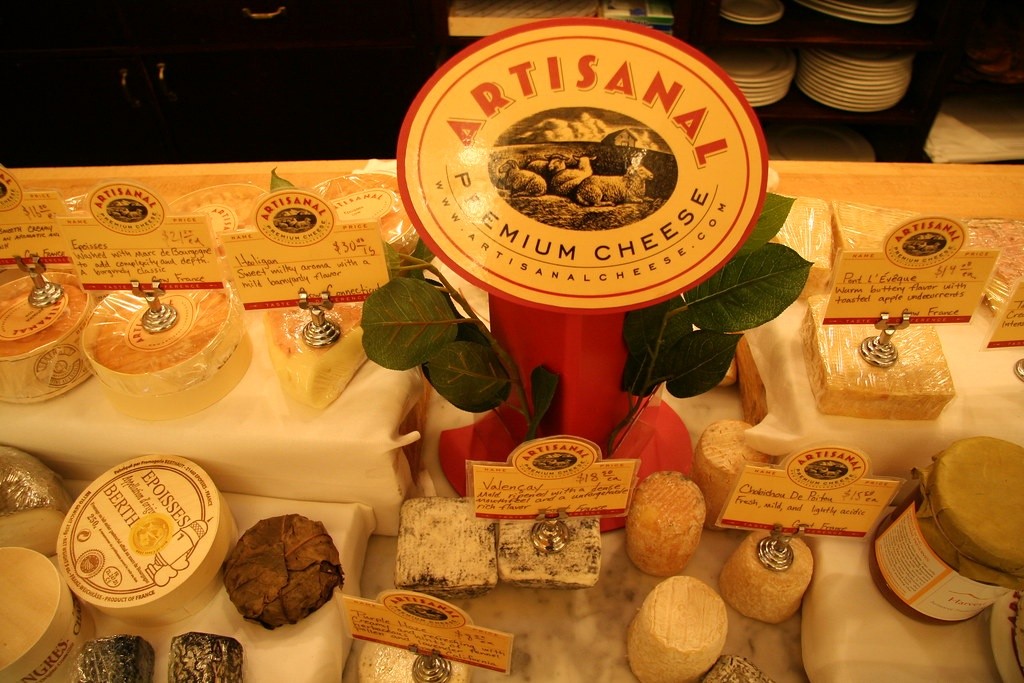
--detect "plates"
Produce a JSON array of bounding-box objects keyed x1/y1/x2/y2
[
  {"x1": 794, "y1": 44, "x2": 914, "y2": 113},
  {"x1": 700, "y1": 42, "x2": 795, "y2": 108},
  {"x1": 719, "y1": 0, "x2": 784, "y2": 25},
  {"x1": 794, "y1": 0, "x2": 916, "y2": 25}
]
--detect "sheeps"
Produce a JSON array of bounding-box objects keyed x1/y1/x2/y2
[
  {"x1": 526, "y1": 154, "x2": 569, "y2": 180},
  {"x1": 577, "y1": 166, "x2": 654, "y2": 206},
  {"x1": 497, "y1": 160, "x2": 547, "y2": 197},
  {"x1": 550, "y1": 155, "x2": 598, "y2": 194}
]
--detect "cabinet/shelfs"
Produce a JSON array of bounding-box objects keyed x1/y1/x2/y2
[{"x1": 0, "y1": 1, "x2": 1023, "y2": 164}]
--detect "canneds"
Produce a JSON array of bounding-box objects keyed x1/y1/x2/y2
[{"x1": 868, "y1": 434, "x2": 1024, "y2": 623}]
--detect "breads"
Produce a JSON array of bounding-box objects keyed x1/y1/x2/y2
[{"x1": 0, "y1": 171, "x2": 1024, "y2": 683}]
